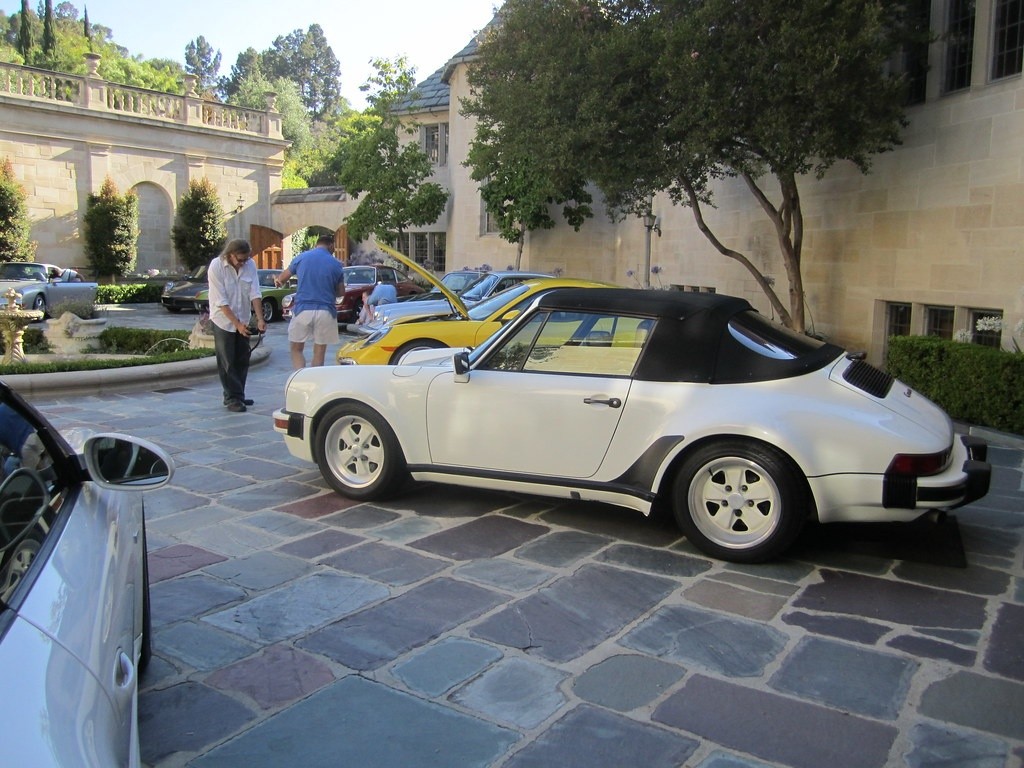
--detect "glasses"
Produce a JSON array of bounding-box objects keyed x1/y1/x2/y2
[{"x1": 235, "y1": 255, "x2": 248, "y2": 263}]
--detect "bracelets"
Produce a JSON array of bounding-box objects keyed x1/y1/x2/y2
[{"x1": 257, "y1": 317, "x2": 263, "y2": 320}]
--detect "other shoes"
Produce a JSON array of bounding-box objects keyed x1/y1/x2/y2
[
  {"x1": 242, "y1": 400, "x2": 254, "y2": 405},
  {"x1": 223, "y1": 399, "x2": 247, "y2": 412}
]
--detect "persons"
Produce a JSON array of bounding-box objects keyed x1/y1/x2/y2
[
  {"x1": 208, "y1": 240, "x2": 267, "y2": 412},
  {"x1": 52, "y1": 269, "x2": 85, "y2": 283},
  {"x1": 355, "y1": 281, "x2": 397, "y2": 326},
  {"x1": 277, "y1": 235, "x2": 345, "y2": 370}
]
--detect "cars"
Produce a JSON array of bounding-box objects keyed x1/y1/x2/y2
[
  {"x1": 194, "y1": 268, "x2": 296, "y2": 322},
  {"x1": 335, "y1": 238, "x2": 627, "y2": 366},
  {"x1": 1, "y1": 261, "x2": 100, "y2": 321},
  {"x1": 160, "y1": 265, "x2": 210, "y2": 311},
  {"x1": 359, "y1": 271, "x2": 560, "y2": 332},
  {"x1": 414, "y1": 266, "x2": 485, "y2": 301},
  {"x1": 280, "y1": 264, "x2": 427, "y2": 326},
  {"x1": 1, "y1": 380, "x2": 176, "y2": 766},
  {"x1": 273, "y1": 285, "x2": 993, "y2": 563}
]
